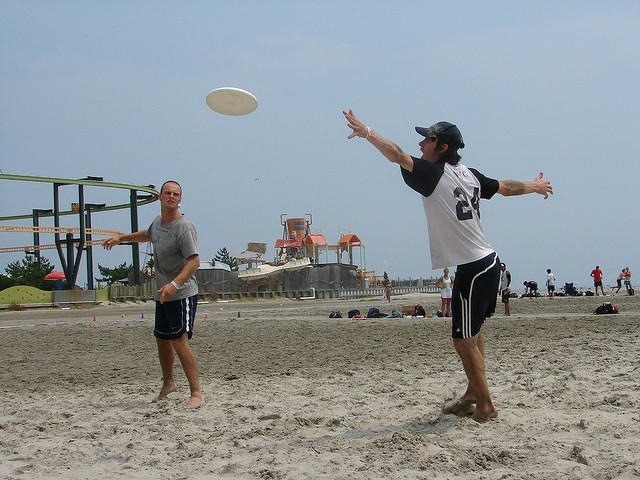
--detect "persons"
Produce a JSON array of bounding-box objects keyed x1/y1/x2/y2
[
  {"x1": 101, "y1": 181, "x2": 205, "y2": 409},
  {"x1": 624, "y1": 267, "x2": 634, "y2": 295},
  {"x1": 590, "y1": 266, "x2": 606, "y2": 296},
  {"x1": 343, "y1": 109, "x2": 553, "y2": 423},
  {"x1": 523, "y1": 280, "x2": 538, "y2": 299},
  {"x1": 385, "y1": 280, "x2": 392, "y2": 303},
  {"x1": 610, "y1": 269, "x2": 625, "y2": 293},
  {"x1": 546, "y1": 268, "x2": 556, "y2": 299},
  {"x1": 435, "y1": 268, "x2": 454, "y2": 317},
  {"x1": 499, "y1": 263, "x2": 511, "y2": 316}
]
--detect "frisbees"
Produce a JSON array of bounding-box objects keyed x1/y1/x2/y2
[{"x1": 206, "y1": 87, "x2": 259, "y2": 117}]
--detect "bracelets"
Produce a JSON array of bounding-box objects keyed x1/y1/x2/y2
[
  {"x1": 171, "y1": 280, "x2": 179, "y2": 290},
  {"x1": 365, "y1": 125, "x2": 371, "y2": 139}
]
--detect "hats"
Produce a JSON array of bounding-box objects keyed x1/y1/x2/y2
[{"x1": 415, "y1": 122, "x2": 463, "y2": 150}]
